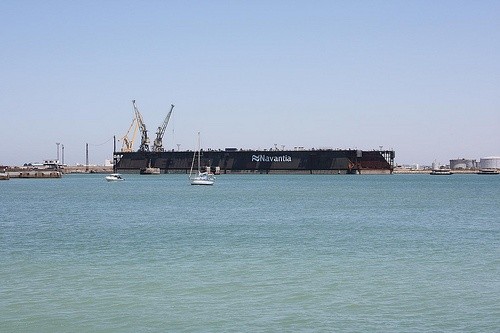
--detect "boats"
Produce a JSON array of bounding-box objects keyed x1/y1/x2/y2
[
  {"x1": 476, "y1": 168, "x2": 500, "y2": 174},
  {"x1": 430, "y1": 169, "x2": 453, "y2": 175},
  {"x1": 104, "y1": 173, "x2": 125, "y2": 183},
  {"x1": 199, "y1": 171, "x2": 216, "y2": 178},
  {"x1": 191, "y1": 178, "x2": 215, "y2": 185}
]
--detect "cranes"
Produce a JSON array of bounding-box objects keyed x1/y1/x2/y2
[
  {"x1": 118, "y1": 113, "x2": 143, "y2": 152},
  {"x1": 151, "y1": 104, "x2": 175, "y2": 152},
  {"x1": 131, "y1": 100, "x2": 151, "y2": 152}
]
